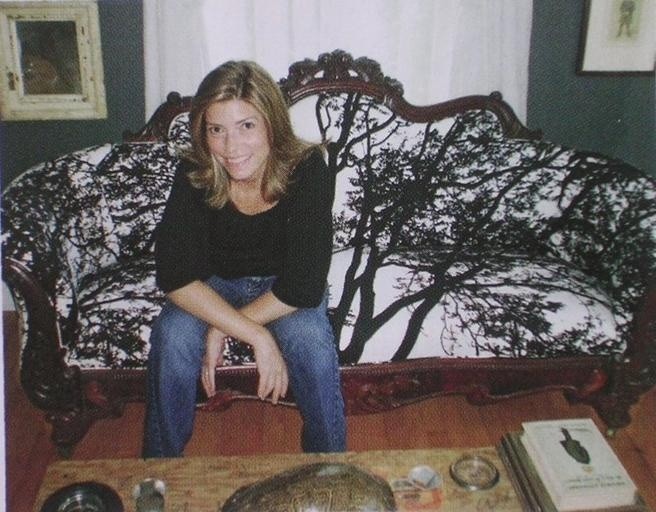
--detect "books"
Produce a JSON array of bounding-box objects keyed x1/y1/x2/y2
[{"x1": 495, "y1": 417, "x2": 654, "y2": 511}]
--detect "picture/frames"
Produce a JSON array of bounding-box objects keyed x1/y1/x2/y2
[
  {"x1": 575, "y1": 0, "x2": 656, "y2": 76},
  {"x1": 0, "y1": 0, "x2": 108, "y2": 123}
]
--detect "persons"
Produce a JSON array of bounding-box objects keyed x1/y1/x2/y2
[{"x1": 139, "y1": 59, "x2": 349, "y2": 459}]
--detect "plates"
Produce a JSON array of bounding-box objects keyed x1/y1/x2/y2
[{"x1": 449, "y1": 453, "x2": 499, "y2": 490}]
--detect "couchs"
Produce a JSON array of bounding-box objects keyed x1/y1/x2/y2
[{"x1": 0, "y1": 51, "x2": 656, "y2": 460}]
